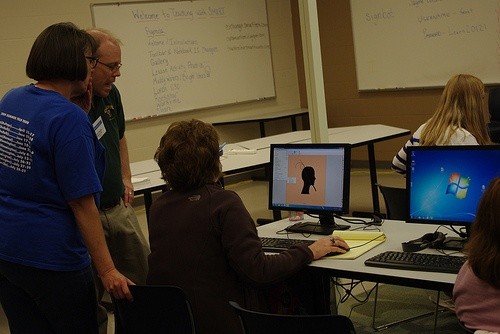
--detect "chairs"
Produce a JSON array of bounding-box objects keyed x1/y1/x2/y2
[
  {"x1": 110, "y1": 285, "x2": 196, "y2": 334},
  {"x1": 228, "y1": 301, "x2": 356, "y2": 334},
  {"x1": 375, "y1": 183, "x2": 408, "y2": 221}
]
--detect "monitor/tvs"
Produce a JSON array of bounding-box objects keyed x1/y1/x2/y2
[
  {"x1": 268, "y1": 144, "x2": 351, "y2": 235},
  {"x1": 405, "y1": 144, "x2": 500, "y2": 250}
]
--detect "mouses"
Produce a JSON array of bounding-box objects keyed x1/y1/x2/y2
[{"x1": 325, "y1": 245, "x2": 350, "y2": 257}]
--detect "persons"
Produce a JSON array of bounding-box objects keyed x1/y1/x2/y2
[
  {"x1": 392, "y1": 75, "x2": 491, "y2": 312},
  {"x1": 89, "y1": 31, "x2": 151, "y2": 334},
  {"x1": 452, "y1": 177, "x2": 500, "y2": 334},
  {"x1": 0, "y1": 23, "x2": 136, "y2": 334},
  {"x1": 147, "y1": 119, "x2": 349, "y2": 334}
]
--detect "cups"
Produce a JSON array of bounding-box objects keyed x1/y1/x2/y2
[{"x1": 288, "y1": 211, "x2": 304, "y2": 221}]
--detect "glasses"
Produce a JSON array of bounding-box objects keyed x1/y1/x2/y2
[
  {"x1": 217, "y1": 147, "x2": 223, "y2": 156},
  {"x1": 85, "y1": 55, "x2": 99, "y2": 69},
  {"x1": 96, "y1": 60, "x2": 122, "y2": 73}
]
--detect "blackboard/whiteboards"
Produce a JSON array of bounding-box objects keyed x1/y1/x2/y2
[
  {"x1": 91, "y1": 0, "x2": 278, "y2": 122},
  {"x1": 350, "y1": 0, "x2": 500, "y2": 93}
]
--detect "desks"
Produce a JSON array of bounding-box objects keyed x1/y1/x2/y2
[
  {"x1": 212, "y1": 107, "x2": 309, "y2": 180},
  {"x1": 255, "y1": 214, "x2": 468, "y2": 334},
  {"x1": 129, "y1": 124, "x2": 411, "y2": 224}
]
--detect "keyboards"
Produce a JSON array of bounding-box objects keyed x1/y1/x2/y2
[
  {"x1": 258, "y1": 236, "x2": 316, "y2": 254},
  {"x1": 364, "y1": 250, "x2": 467, "y2": 274}
]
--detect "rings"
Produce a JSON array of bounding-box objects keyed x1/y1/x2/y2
[
  {"x1": 330, "y1": 238, "x2": 334, "y2": 243},
  {"x1": 128, "y1": 191, "x2": 132, "y2": 195}
]
